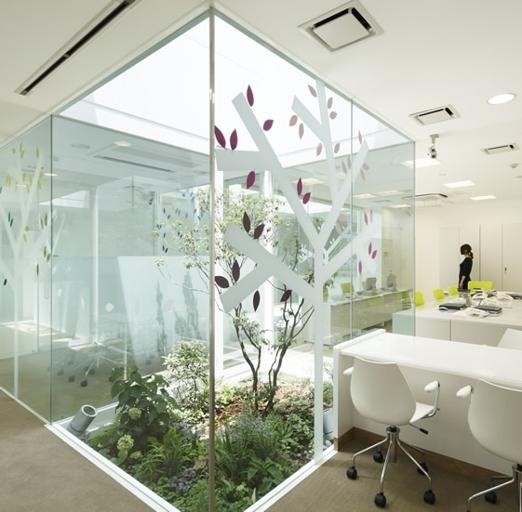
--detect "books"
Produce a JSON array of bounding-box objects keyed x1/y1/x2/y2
[{"x1": 440, "y1": 298, "x2": 500, "y2": 319}]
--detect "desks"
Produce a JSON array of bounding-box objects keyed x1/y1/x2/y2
[
  {"x1": 303, "y1": 288, "x2": 522, "y2": 351},
  {"x1": 332, "y1": 328, "x2": 522, "y2": 477}
]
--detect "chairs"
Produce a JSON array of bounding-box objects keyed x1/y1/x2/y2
[{"x1": 343, "y1": 355, "x2": 522, "y2": 511}]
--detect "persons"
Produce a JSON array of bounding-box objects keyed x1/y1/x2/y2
[{"x1": 458, "y1": 244, "x2": 475, "y2": 289}]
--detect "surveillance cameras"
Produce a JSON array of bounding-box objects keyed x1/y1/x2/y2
[{"x1": 427, "y1": 143, "x2": 439, "y2": 159}]
